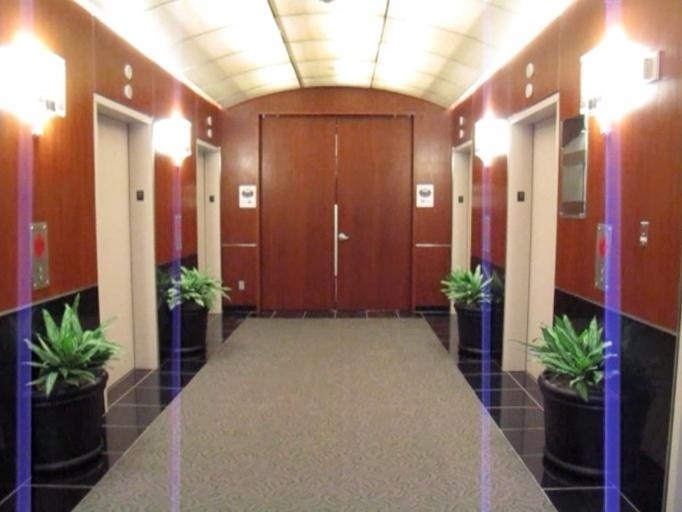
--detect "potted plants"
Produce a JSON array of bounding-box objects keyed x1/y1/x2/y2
[
  {"x1": 440, "y1": 263, "x2": 495, "y2": 360},
  {"x1": 168, "y1": 265, "x2": 232, "y2": 362},
  {"x1": 19, "y1": 293, "x2": 123, "y2": 481},
  {"x1": 508, "y1": 313, "x2": 627, "y2": 487}
]
